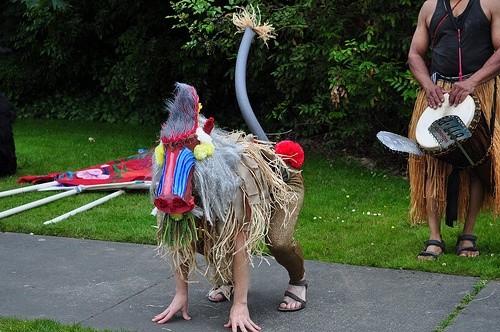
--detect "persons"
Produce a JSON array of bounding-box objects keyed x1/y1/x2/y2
[
  {"x1": 406, "y1": 0, "x2": 500, "y2": 260},
  {"x1": 149, "y1": 3, "x2": 308, "y2": 332}
]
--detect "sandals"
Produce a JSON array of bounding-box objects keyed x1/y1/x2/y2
[
  {"x1": 417, "y1": 234, "x2": 447, "y2": 261},
  {"x1": 455, "y1": 234, "x2": 480, "y2": 258},
  {"x1": 208, "y1": 279, "x2": 234, "y2": 302},
  {"x1": 277, "y1": 272, "x2": 308, "y2": 312}
]
